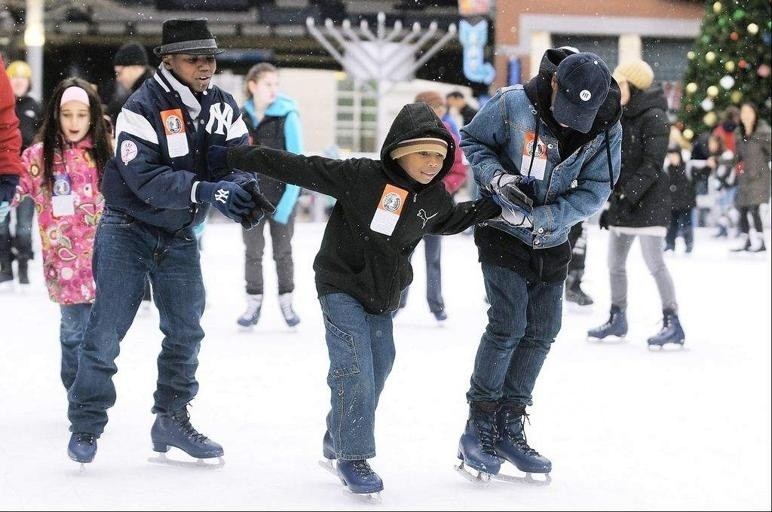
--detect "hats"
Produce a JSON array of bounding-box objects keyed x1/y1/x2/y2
[
  {"x1": 611, "y1": 58, "x2": 652, "y2": 94},
  {"x1": 113, "y1": 40, "x2": 149, "y2": 67},
  {"x1": 152, "y1": 18, "x2": 226, "y2": 55},
  {"x1": 387, "y1": 108, "x2": 452, "y2": 159},
  {"x1": 548, "y1": 52, "x2": 612, "y2": 133}
]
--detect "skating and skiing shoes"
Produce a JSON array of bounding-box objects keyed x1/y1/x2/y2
[
  {"x1": 65, "y1": 430, "x2": 99, "y2": 473},
  {"x1": 497, "y1": 398, "x2": 553, "y2": 489},
  {"x1": 0, "y1": 263, "x2": 13, "y2": 289},
  {"x1": 645, "y1": 317, "x2": 687, "y2": 353},
  {"x1": 18, "y1": 264, "x2": 34, "y2": 290},
  {"x1": 238, "y1": 293, "x2": 263, "y2": 331},
  {"x1": 749, "y1": 232, "x2": 767, "y2": 253},
  {"x1": 147, "y1": 409, "x2": 226, "y2": 474},
  {"x1": 333, "y1": 454, "x2": 386, "y2": 507},
  {"x1": 277, "y1": 293, "x2": 302, "y2": 329},
  {"x1": 453, "y1": 398, "x2": 504, "y2": 485},
  {"x1": 585, "y1": 311, "x2": 629, "y2": 341},
  {"x1": 322, "y1": 431, "x2": 337, "y2": 457},
  {"x1": 732, "y1": 233, "x2": 751, "y2": 252},
  {"x1": 564, "y1": 276, "x2": 593, "y2": 307},
  {"x1": 433, "y1": 310, "x2": 449, "y2": 330}
]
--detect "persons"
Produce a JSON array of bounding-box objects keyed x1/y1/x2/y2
[
  {"x1": 206, "y1": 101, "x2": 538, "y2": 498},
  {"x1": 0, "y1": 77, "x2": 116, "y2": 390},
  {"x1": 720, "y1": 102, "x2": 772, "y2": 252},
  {"x1": 454, "y1": 48, "x2": 621, "y2": 487},
  {"x1": 667, "y1": 116, "x2": 694, "y2": 152},
  {"x1": 666, "y1": 143, "x2": 698, "y2": 253},
  {"x1": 393, "y1": 91, "x2": 469, "y2": 321},
  {"x1": 67, "y1": 15, "x2": 275, "y2": 467},
  {"x1": 237, "y1": 63, "x2": 305, "y2": 327},
  {"x1": 587, "y1": 58, "x2": 689, "y2": 353},
  {"x1": 713, "y1": 106, "x2": 742, "y2": 153},
  {"x1": 0, "y1": 60, "x2": 43, "y2": 283},
  {"x1": 114, "y1": 42, "x2": 156, "y2": 92},
  {"x1": 0, "y1": 54, "x2": 21, "y2": 204},
  {"x1": 688, "y1": 132, "x2": 725, "y2": 226},
  {"x1": 446, "y1": 92, "x2": 477, "y2": 125}
]
